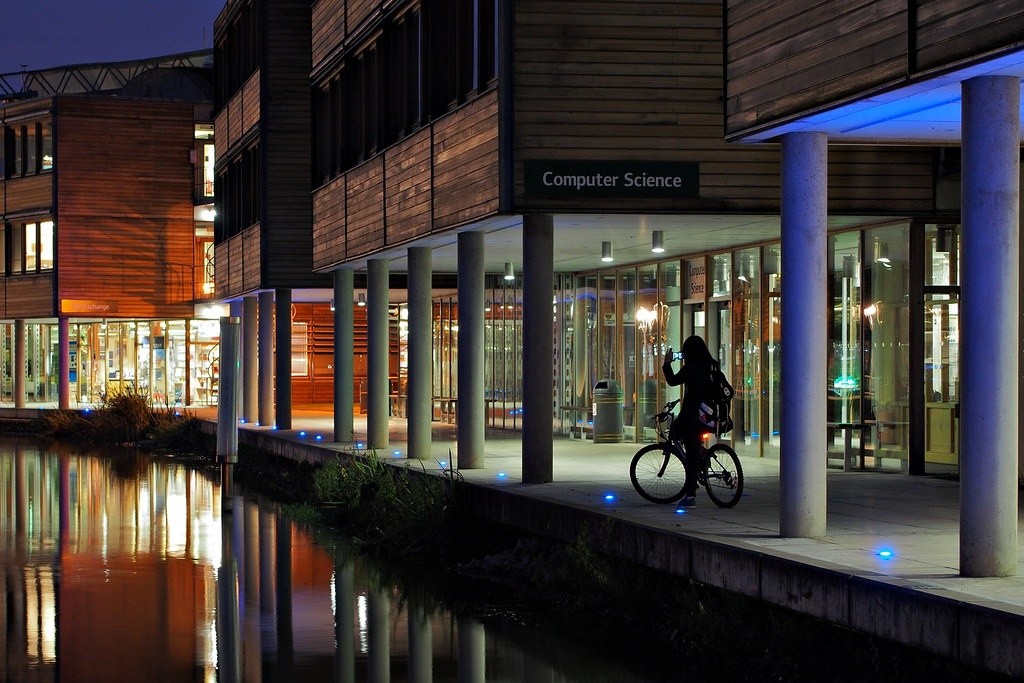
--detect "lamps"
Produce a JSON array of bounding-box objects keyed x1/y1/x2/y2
[
  {"x1": 330, "y1": 300, "x2": 335, "y2": 311},
  {"x1": 507, "y1": 298, "x2": 513, "y2": 308},
  {"x1": 741, "y1": 252, "x2": 754, "y2": 279},
  {"x1": 498, "y1": 322, "x2": 503, "y2": 330},
  {"x1": 601, "y1": 242, "x2": 613, "y2": 262},
  {"x1": 651, "y1": 231, "x2": 665, "y2": 253},
  {"x1": 485, "y1": 300, "x2": 491, "y2": 311},
  {"x1": 486, "y1": 320, "x2": 491, "y2": 328},
  {"x1": 103, "y1": 319, "x2": 107, "y2": 325},
  {"x1": 842, "y1": 256, "x2": 857, "y2": 278},
  {"x1": 935, "y1": 225, "x2": 952, "y2": 252},
  {"x1": 768, "y1": 248, "x2": 780, "y2": 275},
  {"x1": 714, "y1": 256, "x2": 727, "y2": 281},
  {"x1": 358, "y1": 293, "x2": 365, "y2": 306},
  {"x1": 504, "y1": 263, "x2": 515, "y2": 280},
  {"x1": 876, "y1": 242, "x2": 889, "y2": 261}
]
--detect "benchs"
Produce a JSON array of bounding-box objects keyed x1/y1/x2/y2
[
  {"x1": 827, "y1": 422, "x2": 862, "y2": 471},
  {"x1": 433, "y1": 399, "x2": 491, "y2": 425},
  {"x1": 865, "y1": 420, "x2": 908, "y2": 469}
]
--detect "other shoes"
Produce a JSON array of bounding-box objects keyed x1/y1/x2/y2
[
  {"x1": 698, "y1": 459, "x2": 711, "y2": 472},
  {"x1": 677, "y1": 497, "x2": 696, "y2": 509}
]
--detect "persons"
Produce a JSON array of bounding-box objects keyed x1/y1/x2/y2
[{"x1": 662, "y1": 335, "x2": 721, "y2": 509}]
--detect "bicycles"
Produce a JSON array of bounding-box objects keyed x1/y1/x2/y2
[{"x1": 628, "y1": 398, "x2": 745, "y2": 510}]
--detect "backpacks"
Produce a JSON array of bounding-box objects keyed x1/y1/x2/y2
[{"x1": 697, "y1": 369, "x2": 730, "y2": 428}]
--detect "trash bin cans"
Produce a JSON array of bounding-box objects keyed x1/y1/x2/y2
[{"x1": 591, "y1": 378, "x2": 624, "y2": 442}]
